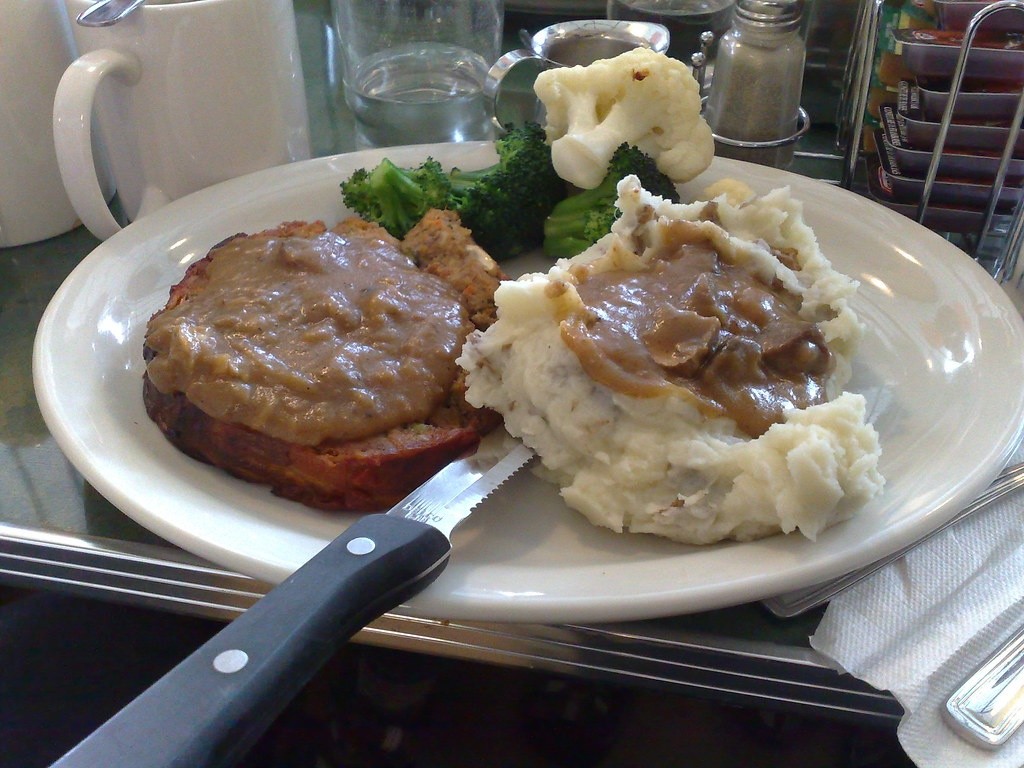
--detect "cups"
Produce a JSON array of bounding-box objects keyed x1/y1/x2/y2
[
  {"x1": 334, "y1": 0, "x2": 503, "y2": 150},
  {"x1": 0, "y1": 0, "x2": 116, "y2": 247},
  {"x1": 51, "y1": 0, "x2": 312, "y2": 240}
]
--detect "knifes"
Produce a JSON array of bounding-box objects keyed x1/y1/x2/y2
[{"x1": 49, "y1": 420, "x2": 538, "y2": 768}]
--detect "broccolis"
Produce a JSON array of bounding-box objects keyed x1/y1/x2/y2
[{"x1": 341, "y1": 47, "x2": 715, "y2": 259}]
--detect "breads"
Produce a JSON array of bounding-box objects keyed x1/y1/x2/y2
[{"x1": 140, "y1": 207, "x2": 512, "y2": 509}]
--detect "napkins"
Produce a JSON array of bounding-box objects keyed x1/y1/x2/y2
[{"x1": 806, "y1": 437, "x2": 1024, "y2": 768}]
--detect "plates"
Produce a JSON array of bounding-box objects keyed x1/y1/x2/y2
[{"x1": 33, "y1": 143, "x2": 1024, "y2": 622}]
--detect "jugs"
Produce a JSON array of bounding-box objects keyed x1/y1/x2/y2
[{"x1": 486, "y1": 19, "x2": 670, "y2": 131}]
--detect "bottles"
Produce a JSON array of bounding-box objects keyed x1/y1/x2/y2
[{"x1": 702, "y1": 1, "x2": 806, "y2": 142}]
